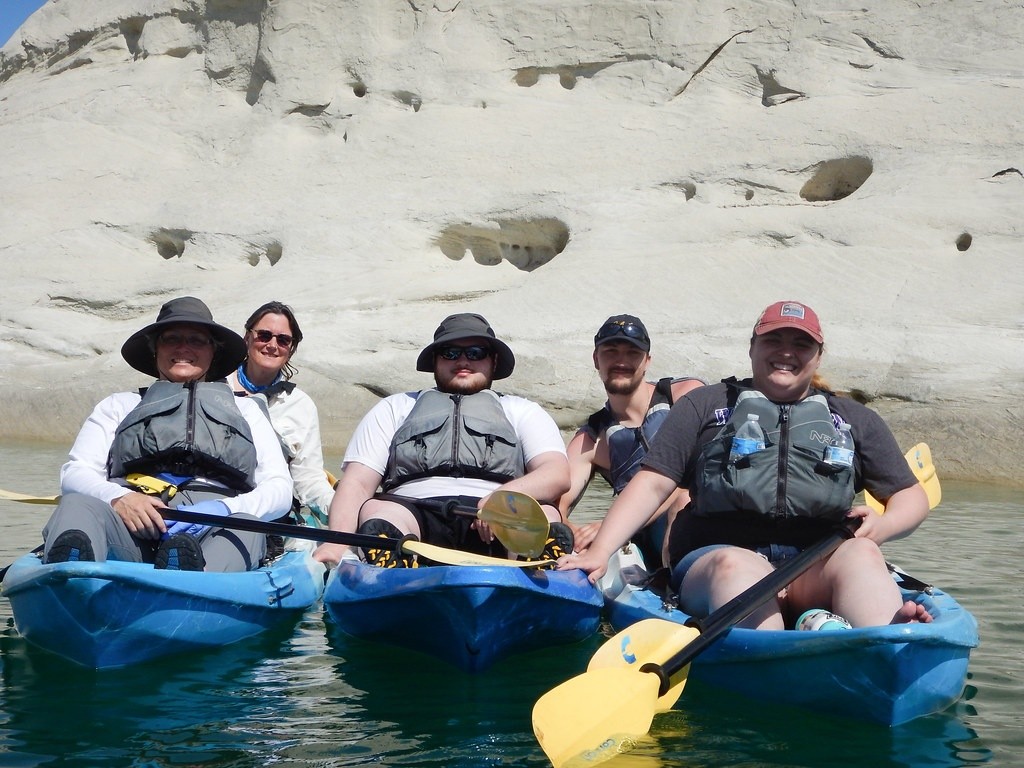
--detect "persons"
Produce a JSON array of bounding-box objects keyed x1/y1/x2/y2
[
  {"x1": 555, "y1": 313, "x2": 708, "y2": 575},
  {"x1": 41, "y1": 295, "x2": 293, "y2": 572},
  {"x1": 553, "y1": 300, "x2": 932, "y2": 632},
  {"x1": 312, "y1": 313, "x2": 576, "y2": 570},
  {"x1": 225, "y1": 301, "x2": 337, "y2": 525}
]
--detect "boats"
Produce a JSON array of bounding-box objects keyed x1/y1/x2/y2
[
  {"x1": 595, "y1": 542, "x2": 979, "y2": 728},
  {"x1": 321, "y1": 541, "x2": 606, "y2": 703},
  {"x1": 0, "y1": 511, "x2": 332, "y2": 685}
]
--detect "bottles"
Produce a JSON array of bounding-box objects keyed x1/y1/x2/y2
[
  {"x1": 796, "y1": 608, "x2": 852, "y2": 631},
  {"x1": 727, "y1": 413, "x2": 767, "y2": 468},
  {"x1": 822, "y1": 423, "x2": 855, "y2": 467}
]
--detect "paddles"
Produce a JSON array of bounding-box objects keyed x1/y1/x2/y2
[
  {"x1": 0, "y1": 486, "x2": 560, "y2": 570},
  {"x1": 530, "y1": 439, "x2": 944, "y2": 768},
  {"x1": 322, "y1": 465, "x2": 552, "y2": 559}
]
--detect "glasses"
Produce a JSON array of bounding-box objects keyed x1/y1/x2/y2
[
  {"x1": 595, "y1": 322, "x2": 650, "y2": 342},
  {"x1": 434, "y1": 344, "x2": 495, "y2": 361},
  {"x1": 156, "y1": 329, "x2": 215, "y2": 348},
  {"x1": 250, "y1": 329, "x2": 295, "y2": 346}
]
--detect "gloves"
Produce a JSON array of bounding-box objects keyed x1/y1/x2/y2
[{"x1": 164, "y1": 500, "x2": 231, "y2": 539}]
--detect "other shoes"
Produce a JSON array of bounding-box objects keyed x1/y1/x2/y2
[
  {"x1": 154, "y1": 532, "x2": 206, "y2": 571},
  {"x1": 516, "y1": 522, "x2": 574, "y2": 571},
  {"x1": 46, "y1": 529, "x2": 97, "y2": 562},
  {"x1": 358, "y1": 518, "x2": 421, "y2": 568}
]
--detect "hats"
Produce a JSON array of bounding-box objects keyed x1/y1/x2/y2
[
  {"x1": 595, "y1": 314, "x2": 651, "y2": 352},
  {"x1": 121, "y1": 296, "x2": 248, "y2": 382},
  {"x1": 753, "y1": 300, "x2": 823, "y2": 344},
  {"x1": 416, "y1": 313, "x2": 515, "y2": 380}
]
